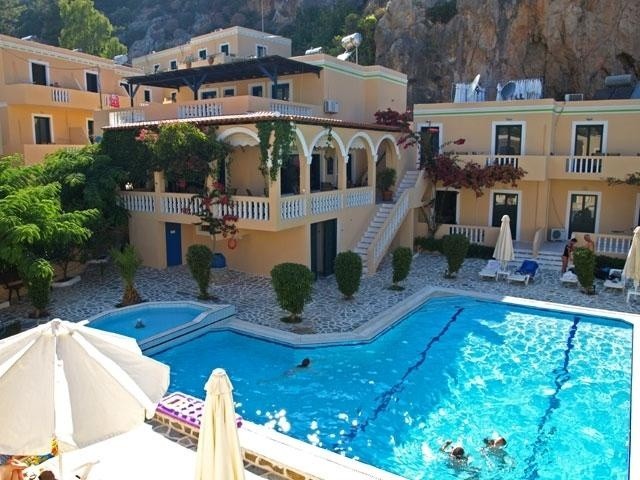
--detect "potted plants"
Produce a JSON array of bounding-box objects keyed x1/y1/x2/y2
[{"x1": 376, "y1": 167, "x2": 397, "y2": 200}]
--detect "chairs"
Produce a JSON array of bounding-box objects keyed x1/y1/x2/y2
[
  {"x1": 477, "y1": 259, "x2": 539, "y2": 286},
  {"x1": 559, "y1": 265, "x2": 625, "y2": 295}
]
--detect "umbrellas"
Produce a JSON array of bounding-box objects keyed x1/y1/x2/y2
[
  {"x1": 134, "y1": 319, "x2": 146, "y2": 328},
  {"x1": 621, "y1": 226, "x2": 640, "y2": 300},
  {"x1": 196, "y1": 368, "x2": 244, "y2": 480},
  {"x1": 493, "y1": 215, "x2": 515, "y2": 270},
  {"x1": 0, "y1": 318, "x2": 170, "y2": 480}
]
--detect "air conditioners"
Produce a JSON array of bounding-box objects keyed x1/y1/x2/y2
[
  {"x1": 324, "y1": 99, "x2": 339, "y2": 115},
  {"x1": 548, "y1": 227, "x2": 566, "y2": 242}
]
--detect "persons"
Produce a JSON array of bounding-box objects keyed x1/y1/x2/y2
[
  {"x1": 581, "y1": 234, "x2": 594, "y2": 251},
  {"x1": 483, "y1": 437, "x2": 507, "y2": 457},
  {"x1": 439, "y1": 441, "x2": 468, "y2": 459},
  {"x1": 562, "y1": 238, "x2": 578, "y2": 273},
  {"x1": 283, "y1": 358, "x2": 310, "y2": 377}
]
